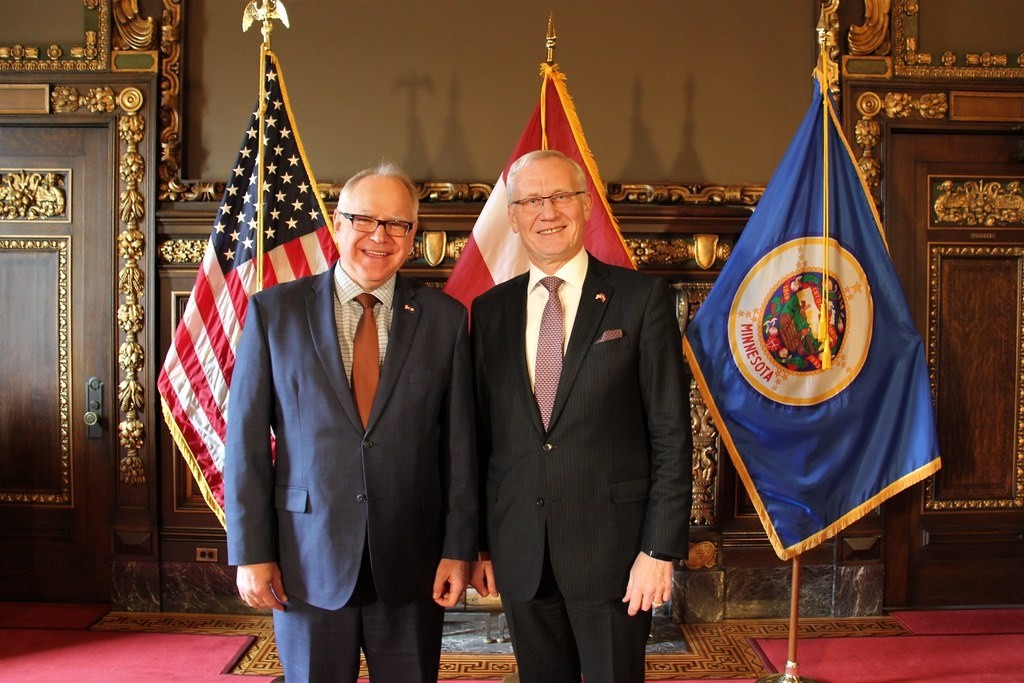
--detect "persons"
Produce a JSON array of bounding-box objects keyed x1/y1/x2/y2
[
  {"x1": 222, "y1": 164, "x2": 486, "y2": 682},
  {"x1": 460, "y1": 147, "x2": 695, "y2": 683}
]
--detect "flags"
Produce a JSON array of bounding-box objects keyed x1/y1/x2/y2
[
  {"x1": 153, "y1": 48, "x2": 344, "y2": 539},
  {"x1": 679, "y1": 68, "x2": 943, "y2": 562},
  {"x1": 442, "y1": 68, "x2": 639, "y2": 333}
]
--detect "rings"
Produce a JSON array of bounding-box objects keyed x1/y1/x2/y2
[{"x1": 651, "y1": 601, "x2": 662, "y2": 608}]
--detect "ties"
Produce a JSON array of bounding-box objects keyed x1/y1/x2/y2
[
  {"x1": 534, "y1": 276, "x2": 564, "y2": 433},
  {"x1": 350, "y1": 293, "x2": 383, "y2": 434}
]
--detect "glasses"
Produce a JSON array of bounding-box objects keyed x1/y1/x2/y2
[
  {"x1": 341, "y1": 213, "x2": 413, "y2": 237},
  {"x1": 512, "y1": 191, "x2": 584, "y2": 213}
]
